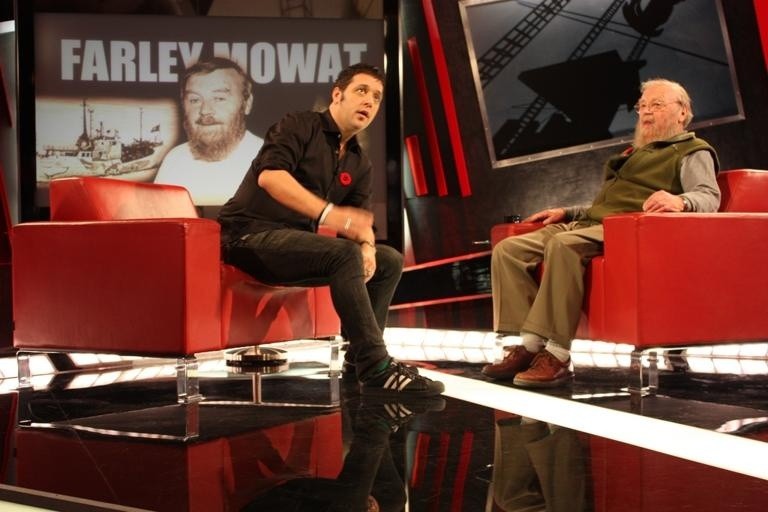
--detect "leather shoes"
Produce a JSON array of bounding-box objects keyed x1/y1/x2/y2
[
  {"x1": 512, "y1": 349, "x2": 575, "y2": 388},
  {"x1": 480, "y1": 344, "x2": 537, "y2": 380}
]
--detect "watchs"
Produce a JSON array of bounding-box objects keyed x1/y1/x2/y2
[
  {"x1": 358, "y1": 239, "x2": 377, "y2": 249},
  {"x1": 679, "y1": 196, "x2": 689, "y2": 213}
]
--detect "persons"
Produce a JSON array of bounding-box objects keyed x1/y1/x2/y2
[
  {"x1": 490, "y1": 411, "x2": 597, "y2": 511},
  {"x1": 219, "y1": 61, "x2": 446, "y2": 398},
  {"x1": 244, "y1": 399, "x2": 444, "y2": 511},
  {"x1": 153, "y1": 55, "x2": 267, "y2": 209},
  {"x1": 480, "y1": 77, "x2": 722, "y2": 390}
]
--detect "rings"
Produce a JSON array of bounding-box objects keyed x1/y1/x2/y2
[{"x1": 343, "y1": 219, "x2": 351, "y2": 229}]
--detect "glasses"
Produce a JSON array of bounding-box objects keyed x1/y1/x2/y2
[{"x1": 633, "y1": 100, "x2": 681, "y2": 114}]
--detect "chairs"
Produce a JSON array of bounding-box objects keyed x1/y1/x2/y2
[
  {"x1": 490, "y1": 168, "x2": 768, "y2": 398},
  {"x1": 8, "y1": 175, "x2": 366, "y2": 445}
]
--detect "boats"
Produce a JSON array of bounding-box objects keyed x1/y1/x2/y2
[{"x1": 35, "y1": 100, "x2": 177, "y2": 187}]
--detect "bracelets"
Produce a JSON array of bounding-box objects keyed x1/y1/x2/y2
[{"x1": 315, "y1": 200, "x2": 334, "y2": 224}]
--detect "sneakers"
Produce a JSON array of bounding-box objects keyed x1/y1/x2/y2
[
  {"x1": 341, "y1": 364, "x2": 417, "y2": 385},
  {"x1": 361, "y1": 397, "x2": 446, "y2": 433},
  {"x1": 360, "y1": 363, "x2": 444, "y2": 397}
]
